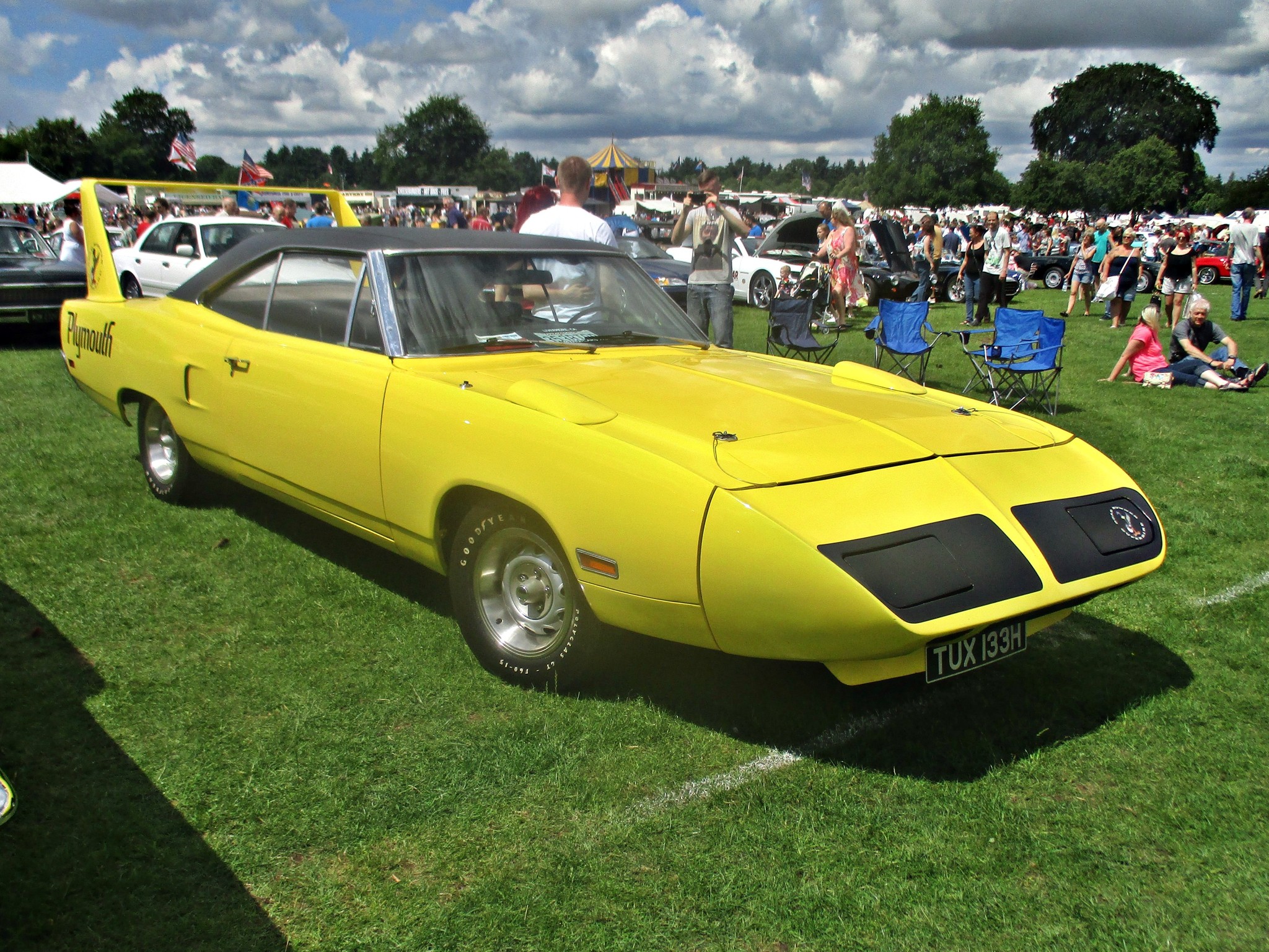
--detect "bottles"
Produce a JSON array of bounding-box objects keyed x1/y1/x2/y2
[
  {"x1": 707, "y1": 201, "x2": 716, "y2": 222},
  {"x1": 1062, "y1": 279, "x2": 1069, "y2": 292}
]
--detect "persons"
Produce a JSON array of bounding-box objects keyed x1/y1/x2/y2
[
  {"x1": 0, "y1": 196, "x2": 517, "y2": 262},
  {"x1": 1060, "y1": 207, "x2": 1269, "y2": 328},
  {"x1": 816, "y1": 200, "x2": 868, "y2": 331},
  {"x1": 508, "y1": 184, "x2": 596, "y2": 313},
  {"x1": 494, "y1": 156, "x2": 622, "y2": 324},
  {"x1": 1097, "y1": 299, "x2": 1269, "y2": 392},
  {"x1": 623, "y1": 209, "x2": 679, "y2": 253},
  {"x1": 774, "y1": 265, "x2": 794, "y2": 299},
  {"x1": 671, "y1": 169, "x2": 750, "y2": 350},
  {"x1": 740, "y1": 208, "x2": 792, "y2": 237},
  {"x1": 860, "y1": 208, "x2": 1070, "y2": 327}
]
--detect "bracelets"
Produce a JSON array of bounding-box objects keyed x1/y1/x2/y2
[{"x1": 1210, "y1": 360, "x2": 1214, "y2": 364}]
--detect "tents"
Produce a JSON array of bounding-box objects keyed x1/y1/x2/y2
[
  {"x1": 0, "y1": 162, "x2": 131, "y2": 210},
  {"x1": 587, "y1": 144, "x2": 655, "y2": 186}
]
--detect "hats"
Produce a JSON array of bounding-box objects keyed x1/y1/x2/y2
[
  {"x1": 1107, "y1": 220, "x2": 1122, "y2": 229},
  {"x1": 1155, "y1": 222, "x2": 1160, "y2": 226}
]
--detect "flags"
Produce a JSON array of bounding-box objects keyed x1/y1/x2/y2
[
  {"x1": 168, "y1": 131, "x2": 198, "y2": 172},
  {"x1": 696, "y1": 159, "x2": 702, "y2": 170},
  {"x1": 802, "y1": 172, "x2": 812, "y2": 191},
  {"x1": 542, "y1": 163, "x2": 555, "y2": 177},
  {"x1": 737, "y1": 168, "x2": 744, "y2": 183},
  {"x1": 238, "y1": 152, "x2": 273, "y2": 210},
  {"x1": 863, "y1": 191, "x2": 868, "y2": 200}
]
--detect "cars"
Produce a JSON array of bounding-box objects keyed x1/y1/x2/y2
[
  {"x1": 107, "y1": 216, "x2": 363, "y2": 303},
  {"x1": 0, "y1": 216, "x2": 88, "y2": 330},
  {"x1": 15, "y1": 225, "x2": 139, "y2": 258},
  {"x1": 1130, "y1": 231, "x2": 1157, "y2": 248},
  {"x1": 1196, "y1": 232, "x2": 1268, "y2": 284},
  {"x1": 610, "y1": 234, "x2": 696, "y2": 316}
]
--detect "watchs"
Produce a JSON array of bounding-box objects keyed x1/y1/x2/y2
[{"x1": 1228, "y1": 355, "x2": 1237, "y2": 359}]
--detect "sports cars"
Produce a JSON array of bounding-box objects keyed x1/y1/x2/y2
[
  {"x1": 863, "y1": 216, "x2": 1022, "y2": 305},
  {"x1": 665, "y1": 209, "x2": 831, "y2": 311},
  {"x1": 59, "y1": 173, "x2": 1168, "y2": 695},
  {"x1": 1027, "y1": 246, "x2": 1167, "y2": 293}
]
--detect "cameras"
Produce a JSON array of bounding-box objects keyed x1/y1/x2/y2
[{"x1": 690, "y1": 191, "x2": 707, "y2": 205}]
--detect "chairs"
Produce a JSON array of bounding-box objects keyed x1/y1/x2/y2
[
  {"x1": 864, "y1": 299, "x2": 953, "y2": 388},
  {"x1": 950, "y1": 306, "x2": 1065, "y2": 419},
  {"x1": 765, "y1": 297, "x2": 853, "y2": 364},
  {"x1": 482, "y1": 301, "x2": 525, "y2": 328}
]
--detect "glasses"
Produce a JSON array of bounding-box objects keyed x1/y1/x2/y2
[
  {"x1": 1176, "y1": 237, "x2": 1187, "y2": 241},
  {"x1": 1123, "y1": 236, "x2": 1132, "y2": 240},
  {"x1": 829, "y1": 216, "x2": 834, "y2": 221}
]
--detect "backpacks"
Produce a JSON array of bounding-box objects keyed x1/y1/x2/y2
[{"x1": 911, "y1": 234, "x2": 928, "y2": 261}]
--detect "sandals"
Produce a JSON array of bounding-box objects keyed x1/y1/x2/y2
[{"x1": 1218, "y1": 361, "x2": 1269, "y2": 391}]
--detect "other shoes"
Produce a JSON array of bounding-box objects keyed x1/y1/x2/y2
[
  {"x1": 1230, "y1": 316, "x2": 1247, "y2": 322},
  {"x1": 927, "y1": 296, "x2": 936, "y2": 303},
  {"x1": 1083, "y1": 313, "x2": 1089, "y2": 316},
  {"x1": 1254, "y1": 288, "x2": 1263, "y2": 299},
  {"x1": 1091, "y1": 295, "x2": 1104, "y2": 302},
  {"x1": 983, "y1": 312, "x2": 990, "y2": 324},
  {"x1": 1099, "y1": 314, "x2": 1126, "y2": 329},
  {"x1": 1060, "y1": 312, "x2": 1069, "y2": 317},
  {"x1": 848, "y1": 313, "x2": 853, "y2": 318},
  {"x1": 1164, "y1": 321, "x2": 1172, "y2": 327},
  {"x1": 961, "y1": 320, "x2": 969, "y2": 324},
  {"x1": 827, "y1": 315, "x2": 839, "y2": 323},
  {"x1": 837, "y1": 325, "x2": 846, "y2": 331},
  {"x1": 966, "y1": 319, "x2": 982, "y2": 327}
]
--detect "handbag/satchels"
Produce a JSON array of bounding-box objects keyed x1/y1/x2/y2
[
  {"x1": 1142, "y1": 371, "x2": 1173, "y2": 390},
  {"x1": 1151, "y1": 288, "x2": 1162, "y2": 309},
  {"x1": 1182, "y1": 290, "x2": 1202, "y2": 319},
  {"x1": 1096, "y1": 275, "x2": 1121, "y2": 300}
]
